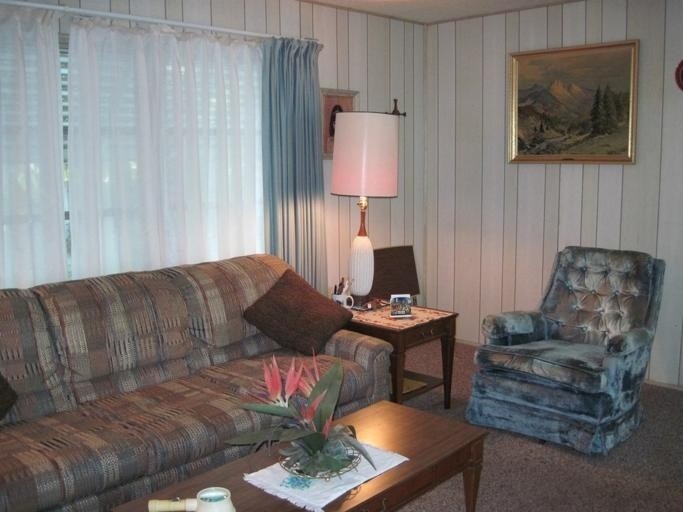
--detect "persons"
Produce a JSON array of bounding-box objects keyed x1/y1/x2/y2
[{"x1": 324, "y1": 104, "x2": 345, "y2": 159}]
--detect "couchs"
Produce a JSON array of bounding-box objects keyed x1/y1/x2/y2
[{"x1": 0, "y1": 252, "x2": 395, "y2": 509}]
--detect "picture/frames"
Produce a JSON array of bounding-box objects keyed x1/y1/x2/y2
[
  {"x1": 319, "y1": 87, "x2": 361, "y2": 162},
  {"x1": 503, "y1": 39, "x2": 639, "y2": 166}
]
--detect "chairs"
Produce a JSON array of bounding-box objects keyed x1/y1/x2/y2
[{"x1": 465, "y1": 246, "x2": 666, "y2": 457}]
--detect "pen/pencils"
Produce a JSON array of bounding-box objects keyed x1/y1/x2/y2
[{"x1": 334, "y1": 277, "x2": 349, "y2": 295}]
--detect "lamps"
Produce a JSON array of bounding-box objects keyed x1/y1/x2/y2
[{"x1": 329, "y1": 110, "x2": 400, "y2": 305}]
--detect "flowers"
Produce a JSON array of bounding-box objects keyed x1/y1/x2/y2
[{"x1": 223, "y1": 347, "x2": 377, "y2": 478}]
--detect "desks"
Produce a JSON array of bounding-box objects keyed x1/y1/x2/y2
[{"x1": 350, "y1": 303, "x2": 459, "y2": 410}]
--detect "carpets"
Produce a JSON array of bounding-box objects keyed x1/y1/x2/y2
[{"x1": 396, "y1": 367, "x2": 439, "y2": 401}]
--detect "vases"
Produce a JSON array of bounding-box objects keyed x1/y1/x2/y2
[{"x1": 277, "y1": 432, "x2": 361, "y2": 480}]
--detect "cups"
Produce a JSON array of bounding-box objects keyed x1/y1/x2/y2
[{"x1": 332, "y1": 295, "x2": 354, "y2": 311}]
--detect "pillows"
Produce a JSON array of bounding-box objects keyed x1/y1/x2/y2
[{"x1": 242, "y1": 267, "x2": 354, "y2": 355}]
